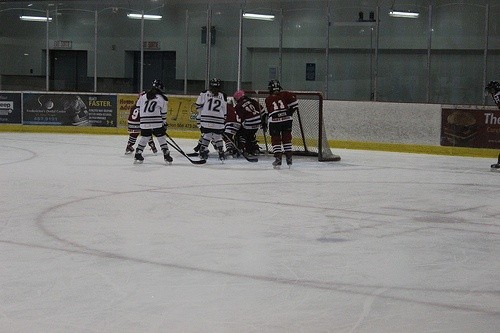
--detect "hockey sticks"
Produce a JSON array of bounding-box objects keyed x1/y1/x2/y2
[
  {"x1": 165, "y1": 140, "x2": 200, "y2": 158},
  {"x1": 222, "y1": 131, "x2": 258, "y2": 163},
  {"x1": 165, "y1": 131, "x2": 207, "y2": 165},
  {"x1": 255, "y1": 88, "x2": 268, "y2": 156}
]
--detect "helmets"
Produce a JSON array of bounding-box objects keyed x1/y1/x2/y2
[
  {"x1": 152, "y1": 79, "x2": 166, "y2": 93},
  {"x1": 210, "y1": 77, "x2": 224, "y2": 92},
  {"x1": 485, "y1": 81, "x2": 500, "y2": 94},
  {"x1": 268, "y1": 79, "x2": 280, "y2": 92},
  {"x1": 227, "y1": 97, "x2": 234, "y2": 104},
  {"x1": 234, "y1": 90, "x2": 245, "y2": 102}
]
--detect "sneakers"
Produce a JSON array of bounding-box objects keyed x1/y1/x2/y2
[
  {"x1": 272, "y1": 156, "x2": 282, "y2": 168},
  {"x1": 152, "y1": 147, "x2": 157, "y2": 156},
  {"x1": 286, "y1": 157, "x2": 292, "y2": 167},
  {"x1": 125, "y1": 145, "x2": 135, "y2": 154},
  {"x1": 251, "y1": 144, "x2": 260, "y2": 156},
  {"x1": 197, "y1": 150, "x2": 209, "y2": 160},
  {"x1": 218, "y1": 151, "x2": 225, "y2": 163},
  {"x1": 223, "y1": 150, "x2": 238, "y2": 160},
  {"x1": 134, "y1": 153, "x2": 144, "y2": 164},
  {"x1": 163, "y1": 154, "x2": 173, "y2": 164},
  {"x1": 237, "y1": 142, "x2": 247, "y2": 157}
]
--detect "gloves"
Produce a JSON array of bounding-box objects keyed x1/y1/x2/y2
[
  {"x1": 260, "y1": 122, "x2": 267, "y2": 129},
  {"x1": 162, "y1": 122, "x2": 168, "y2": 131},
  {"x1": 223, "y1": 132, "x2": 233, "y2": 141}
]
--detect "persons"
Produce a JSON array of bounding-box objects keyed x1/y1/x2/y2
[
  {"x1": 193, "y1": 78, "x2": 268, "y2": 161},
  {"x1": 265, "y1": 79, "x2": 298, "y2": 168},
  {"x1": 485, "y1": 81, "x2": 500, "y2": 172},
  {"x1": 134, "y1": 80, "x2": 174, "y2": 165},
  {"x1": 124, "y1": 91, "x2": 158, "y2": 156}
]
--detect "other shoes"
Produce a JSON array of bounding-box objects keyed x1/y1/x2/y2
[
  {"x1": 490, "y1": 164, "x2": 500, "y2": 172},
  {"x1": 193, "y1": 143, "x2": 202, "y2": 152}
]
